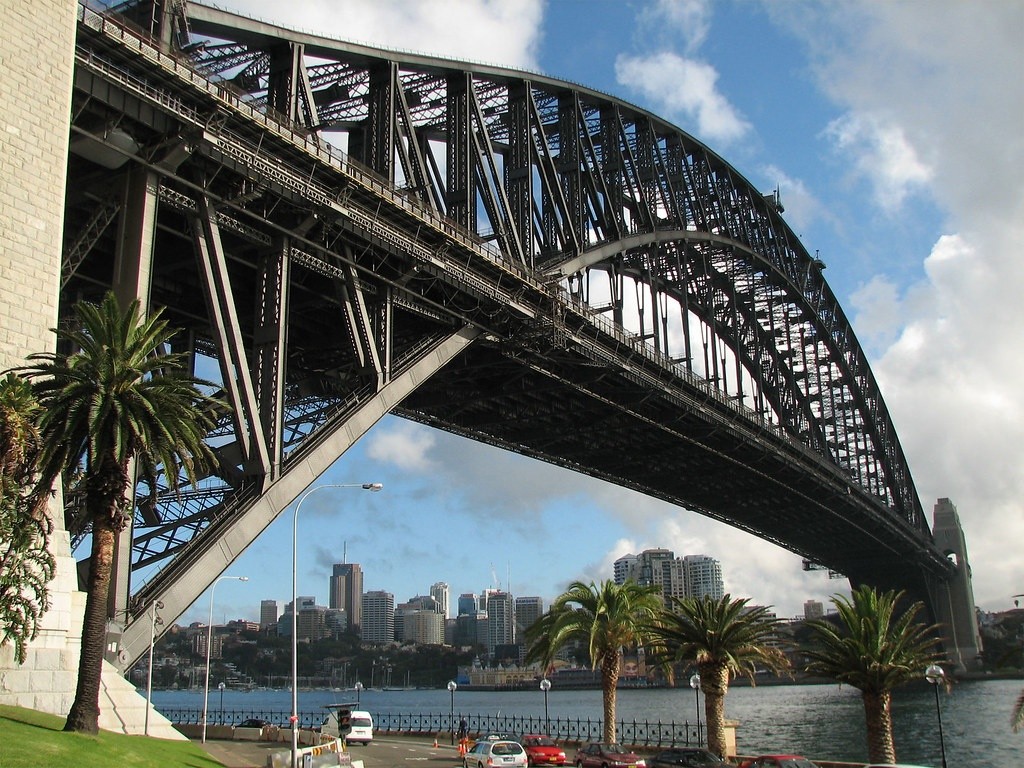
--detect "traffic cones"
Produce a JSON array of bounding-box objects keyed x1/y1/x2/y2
[
  {"x1": 432, "y1": 736, "x2": 439, "y2": 749},
  {"x1": 458, "y1": 736, "x2": 472, "y2": 758}
]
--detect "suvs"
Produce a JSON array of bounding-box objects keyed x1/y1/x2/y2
[{"x1": 319, "y1": 711, "x2": 374, "y2": 746}]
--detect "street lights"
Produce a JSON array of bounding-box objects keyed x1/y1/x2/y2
[
  {"x1": 217, "y1": 682, "x2": 226, "y2": 725},
  {"x1": 287, "y1": 481, "x2": 383, "y2": 768},
  {"x1": 200, "y1": 576, "x2": 249, "y2": 744},
  {"x1": 925, "y1": 663, "x2": 949, "y2": 768},
  {"x1": 143, "y1": 599, "x2": 166, "y2": 734},
  {"x1": 689, "y1": 671, "x2": 702, "y2": 748},
  {"x1": 446, "y1": 680, "x2": 458, "y2": 745},
  {"x1": 538, "y1": 678, "x2": 551, "y2": 736},
  {"x1": 354, "y1": 679, "x2": 363, "y2": 710}
]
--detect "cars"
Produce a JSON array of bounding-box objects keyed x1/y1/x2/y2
[
  {"x1": 647, "y1": 747, "x2": 733, "y2": 768},
  {"x1": 462, "y1": 735, "x2": 529, "y2": 768},
  {"x1": 736, "y1": 753, "x2": 820, "y2": 768},
  {"x1": 572, "y1": 742, "x2": 647, "y2": 768},
  {"x1": 235, "y1": 718, "x2": 272, "y2": 729},
  {"x1": 510, "y1": 734, "x2": 568, "y2": 768},
  {"x1": 475, "y1": 731, "x2": 518, "y2": 741}
]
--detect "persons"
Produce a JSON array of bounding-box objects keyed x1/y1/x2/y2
[{"x1": 459, "y1": 717, "x2": 468, "y2": 739}]
